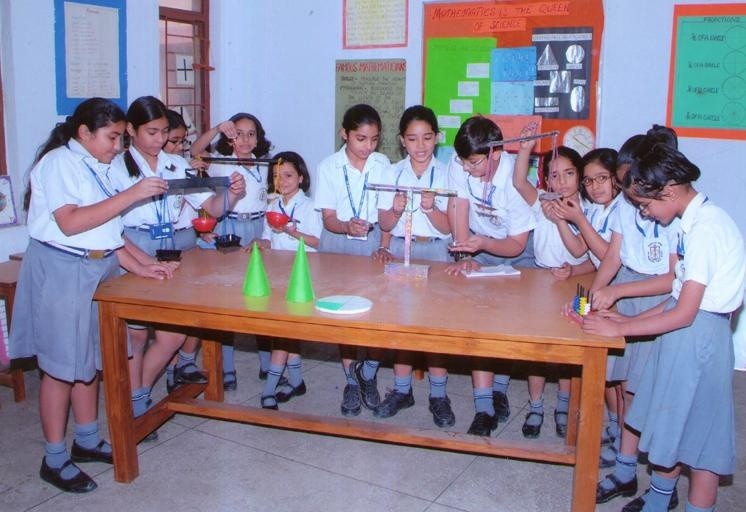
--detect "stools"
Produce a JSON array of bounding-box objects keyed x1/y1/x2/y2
[
  {"x1": 9, "y1": 253, "x2": 26, "y2": 260},
  {"x1": 0, "y1": 261, "x2": 40, "y2": 402}
]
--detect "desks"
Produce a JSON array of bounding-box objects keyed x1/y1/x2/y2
[{"x1": 91, "y1": 247, "x2": 627, "y2": 511}]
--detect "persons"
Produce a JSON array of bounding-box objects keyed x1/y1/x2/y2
[
  {"x1": 313, "y1": 103, "x2": 391, "y2": 417},
  {"x1": 8, "y1": 96, "x2": 173, "y2": 494},
  {"x1": 162, "y1": 108, "x2": 209, "y2": 395},
  {"x1": 189, "y1": 113, "x2": 272, "y2": 390},
  {"x1": 260, "y1": 152, "x2": 324, "y2": 410},
  {"x1": 108, "y1": 96, "x2": 247, "y2": 439}
]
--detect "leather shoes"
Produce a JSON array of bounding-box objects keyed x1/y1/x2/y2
[
  {"x1": 162, "y1": 363, "x2": 209, "y2": 400},
  {"x1": 522, "y1": 408, "x2": 544, "y2": 439},
  {"x1": 338, "y1": 361, "x2": 415, "y2": 418},
  {"x1": 594, "y1": 471, "x2": 638, "y2": 506},
  {"x1": 223, "y1": 369, "x2": 238, "y2": 391},
  {"x1": 620, "y1": 488, "x2": 680, "y2": 512},
  {"x1": 71, "y1": 438, "x2": 116, "y2": 465},
  {"x1": 553, "y1": 407, "x2": 567, "y2": 438},
  {"x1": 599, "y1": 422, "x2": 619, "y2": 467},
  {"x1": 256, "y1": 367, "x2": 306, "y2": 410},
  {"x1": 39, "y1": 455, "x2": 98, "y2": 494},
  {"x1": 467, "y1": 389, "x2": 511, "y2": 436},
  {"x1": 428, "y1": 392, "x2": 455, "y2": 429}
]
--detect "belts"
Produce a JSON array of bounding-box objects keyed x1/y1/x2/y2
[
  {"x1": 125, "y1": 222, "x2": 190, "y2": 235},
  {"x1": 227, "y1": 212, "x2": 264, "y2": 222},
  {"x1": 390, "y1": 234, "x2": 444, "y2": 246},
  {"x1": 31, "y1": 233, "x2": 117, "y2": 261}
]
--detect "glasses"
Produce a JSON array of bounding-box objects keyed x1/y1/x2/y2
[
  {"x1": 454, "y1": 155, "x2": 488, "y2": 170},
  {"x1": 638, "y1": 187, "x2": 666, "y2": 216},
  {"x1": 166, "y1": 137, "x2": 189, "y2": 148},
  {"x1": 580, "y1": 174, "x2": 612, "y2": 186}
]
